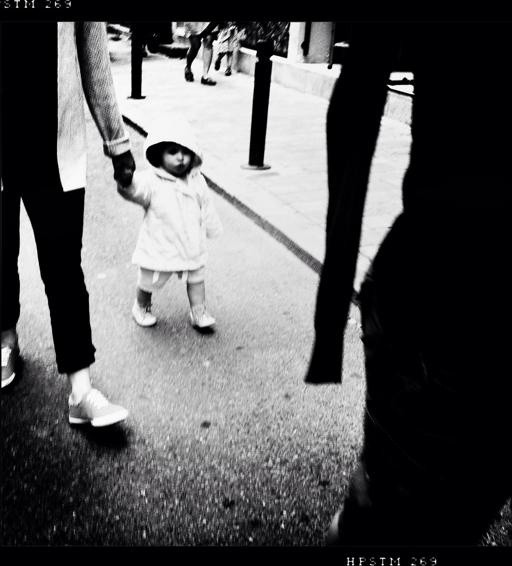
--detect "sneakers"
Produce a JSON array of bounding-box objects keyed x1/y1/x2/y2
[
  {"x1": 69, "y1": 389, "x2": 128, "y2": 428},
  {"x1": 132, "y1": 299, "x2": 157, "y2": 326},
  {"x1": 142, "y1": 41, "x2": 158, "y2": 57},
  {"x1": 189, "y1": 306, "x2": 215, "y2": 328},
  {"x1": 0, "y1": 333, "x2": 19, "y2": 389},
  {"x1": 185, "y1": 59, "x2": 231, "y2": 85}
]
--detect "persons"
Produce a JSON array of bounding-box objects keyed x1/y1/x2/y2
[
  {"x1": 112, "y1": 125, "x2": 218, "y2": 333},
  {"x1": 180, "y1": 19, "x2": 222, "y2": 87},
  {"x1": 300, "y1": 7, "x2": 512, "y2": 551},
  {"x1": 211, "y1": 20, "x2": 241, "y2": 77},
  {"x1": 0, "y1": 20, "x2": 135, "y2": 432}
]
